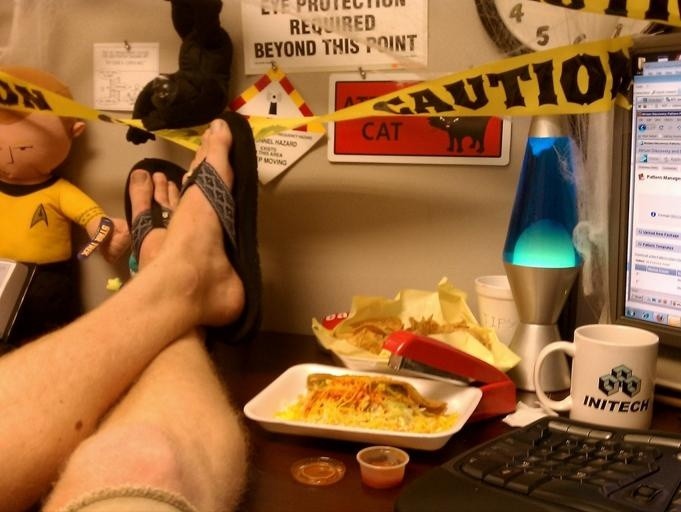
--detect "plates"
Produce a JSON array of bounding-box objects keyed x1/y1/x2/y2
[{"x1": 242, "y1": 363, "x2": 484, "y2": 451}]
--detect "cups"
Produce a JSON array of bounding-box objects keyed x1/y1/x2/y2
[
  {"x1": 476, "y1": 274, "x2": 517, "y2": 349},
  {"x1": 533, "y1": 323, "x2": 660, "y2": 433}
]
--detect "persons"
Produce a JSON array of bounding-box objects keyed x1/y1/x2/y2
[
  {"x1": 0, "y1": 106, "x2": 266, "y2": 512},
  {"x1": 0, "y1": 59, "x2": 135, "y2": 350}
]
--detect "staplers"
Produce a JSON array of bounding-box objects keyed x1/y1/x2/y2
[{"x1": 381, "y1": 330, "x2": 516, "y2": 425}]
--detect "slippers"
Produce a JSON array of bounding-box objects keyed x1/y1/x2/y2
[
  {"x1": 123, "y1": 157, "x2": 186, "y2": 265},
  {"x1": 174, "y1": 109, "x2": 265, "y2": 343}
]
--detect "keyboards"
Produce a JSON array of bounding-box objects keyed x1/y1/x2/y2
[{"x1": 391, "y1": 414, "x2": 681, "y2": 512}]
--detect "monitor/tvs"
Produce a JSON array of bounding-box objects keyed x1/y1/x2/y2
[{"x1": 605, "y1": 31, "x2": 681, "y2": 395}]
